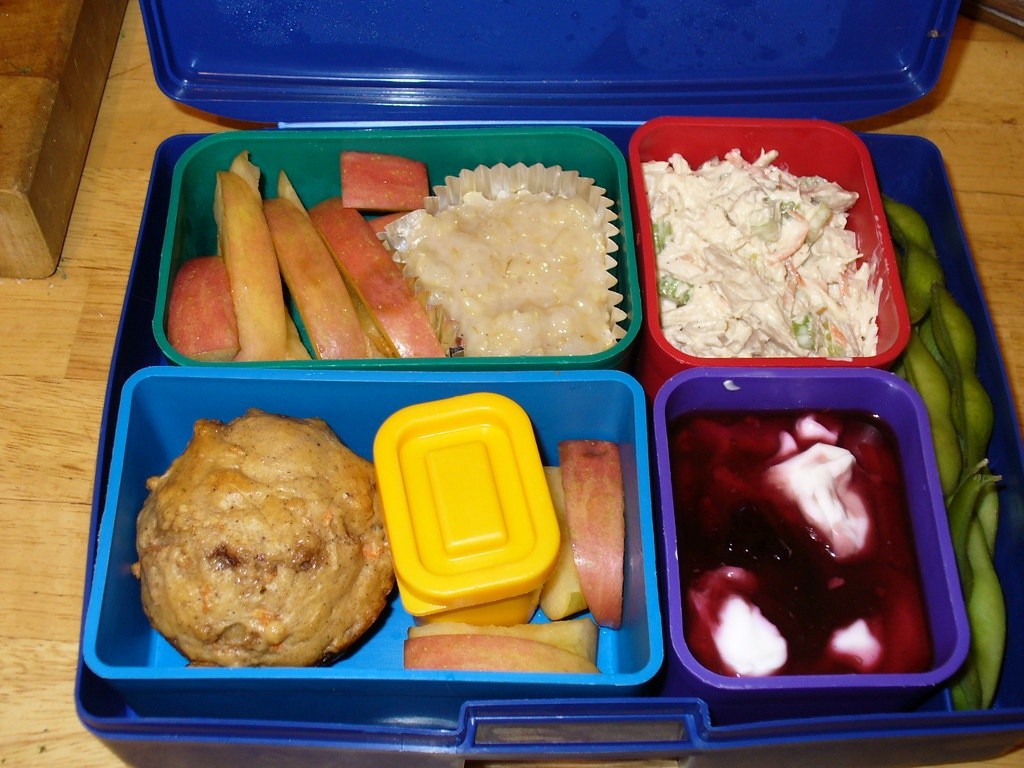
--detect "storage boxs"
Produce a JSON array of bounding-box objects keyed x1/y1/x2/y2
[{"x1": 74, "y1": 0, "x2": 1024, "y2": 767}]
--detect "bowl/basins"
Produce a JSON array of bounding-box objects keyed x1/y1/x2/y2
[
  {"x1": 628, "y1": 116, "x2": 914, "y2": 398},
  {"x1": 150, "y1": 123, "x2": 645, "y2": 369},
  {"x1": 651, "y1": 370, "x2": 970, "y2": 715},
  {"x1": 78, "y1": 366, "x2": 663, "y2": 723}
]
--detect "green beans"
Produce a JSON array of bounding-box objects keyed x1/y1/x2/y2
[{"x1": 877, "y1": 193, "x2": 1011, "y2": 707}]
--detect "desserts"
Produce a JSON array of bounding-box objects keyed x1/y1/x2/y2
[{"x1": 374, "y1": 162, "x2": 628, "y2": 359}]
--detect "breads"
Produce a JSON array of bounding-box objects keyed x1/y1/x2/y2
[{"x1": 132, "y1": 406, "x2": 396, "y2": 668}]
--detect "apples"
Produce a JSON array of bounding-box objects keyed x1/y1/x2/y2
[
  {"x1": 397, "y1": 438, "x2": 622, "y2": 674},
  {"x1": 162, "y1": 149, "x2": 453, "y2": 360}
]
